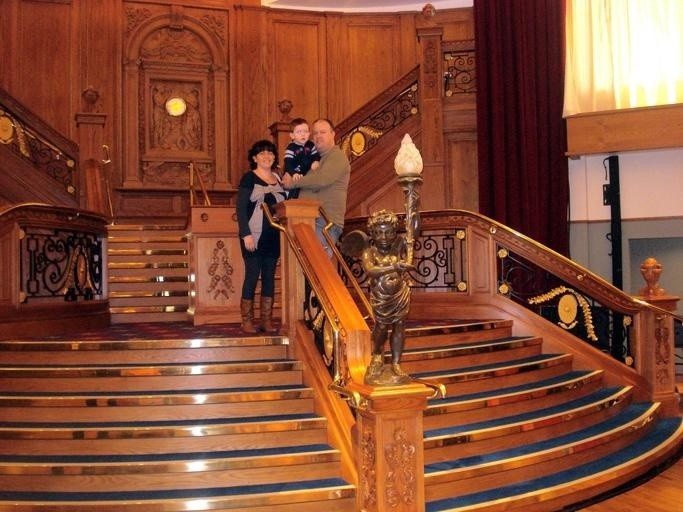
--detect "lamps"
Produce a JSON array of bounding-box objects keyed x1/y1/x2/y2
[{"x1": 361, "y1": 131, "x2": 424, "y2": 387}]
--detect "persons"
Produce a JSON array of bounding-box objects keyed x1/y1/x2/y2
[
  {"x1": 152, "y1": 83, "x2": 170, "y2": 149},
  {"x1": 361, "y1": 207, "x2": 422, "y2": 378},
  {"x1": 237, "y1": 118, "x2": 352, "y2": 335},
  {"x1": 181, "y1": 87, "x2": 202, "y2": 150}
]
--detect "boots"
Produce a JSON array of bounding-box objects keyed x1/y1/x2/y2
[
  {"x1": 260, "y1": 297, "x2": 279, "y2": 333},
  {"x1": 240, "y1": 298, "x2": 259, "y2": 334}
]
